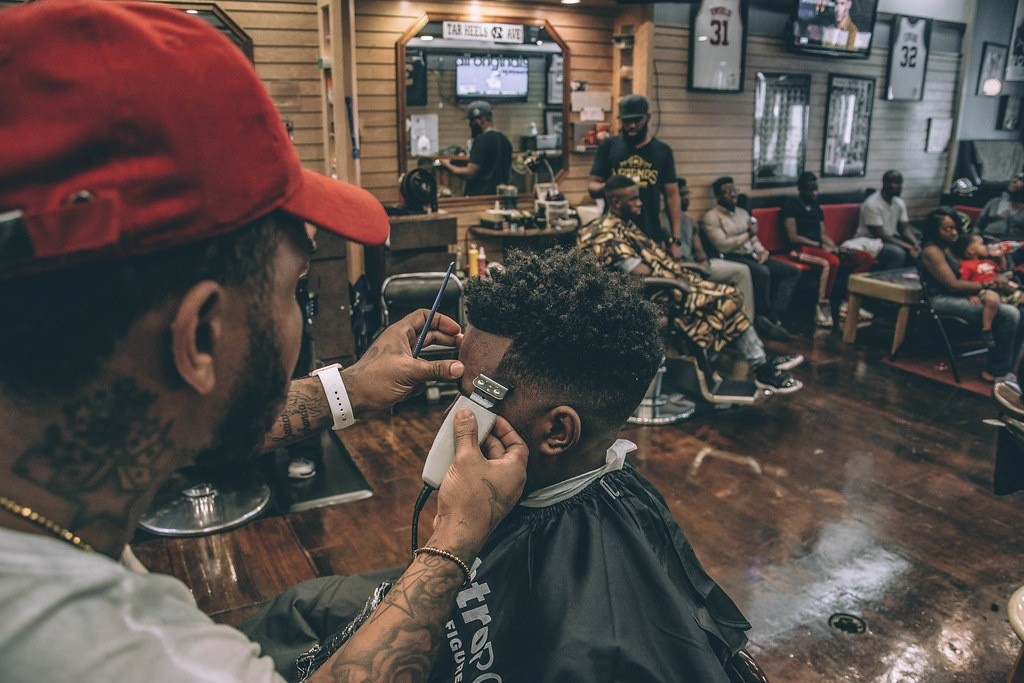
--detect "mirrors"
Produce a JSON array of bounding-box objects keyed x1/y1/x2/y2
[{"x1": 395, "y1": 11, "x2": 571, "y2": 203}]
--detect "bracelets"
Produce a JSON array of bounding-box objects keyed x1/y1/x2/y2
[
  {"x1": 413, "y1": 546, "x2": 472, "y2": 591},
  {"x1": 818, "y1": 242, "x2": 823, "y2": 247},
  {"x1": 308, "y1": 362, "x2": 357, "y2": 431}
]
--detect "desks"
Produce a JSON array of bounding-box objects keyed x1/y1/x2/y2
[
  {"x1": 469, "y1": 213, "x2": 580, "y2": 259},
  {"x1": 429, "y1": 149, "x2": 561, "y2": 199}
]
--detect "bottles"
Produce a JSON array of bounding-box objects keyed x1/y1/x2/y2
[
  {"x1": 455, "y1": 245, "x2": 465, "y2": 279},
  {"x1": 478, "y1": 247, "x2": 487, "y2": 276},
  {"x1": 468, "y1": 243, "x2": 479, "y2": 277},
  {"x1": 988, "y1": 240, "x2": 1024, "y2": 255}
]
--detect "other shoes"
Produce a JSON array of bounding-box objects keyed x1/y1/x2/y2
[
  {"x1": 760, "y1": 314, "x2": 793, "y2": 342},
  {"x1": 839, "y1": 303, "x2": 874, "y2": 324},
  {"x1": 815, "y1": 300, "x2": 834, "y2": 326},
  {"x1": 982, "y1": 370, "x2": 1018, "y2": 384}
]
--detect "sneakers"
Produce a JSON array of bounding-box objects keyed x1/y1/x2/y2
[
  {"x1": 754, "y1": 365, "x2": 803, "y2": 394},
  {"x1": 768, "y1": 351, "x2": 804, "y2": 370}
]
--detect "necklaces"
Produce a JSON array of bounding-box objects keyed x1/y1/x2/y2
[{"x1": 1, "y1": 496, "x2": 96, "y2": 551}]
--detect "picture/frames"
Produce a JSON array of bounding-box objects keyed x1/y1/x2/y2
[
  {"x1": 819, "y1": 73, "x2": 876, "y2": 178},
  {"x1": 881, "y1": 13, "x2": 933, "y2": 102},
  {"x1": 751, "y1": 71, "x2": 811, "y2": 188},
  {"x1": 687, "y1": 0, "x2": 750, "y2": 92},
  {"x1": 977, "y1": 41, "x2": 1010, "y2": 96}
]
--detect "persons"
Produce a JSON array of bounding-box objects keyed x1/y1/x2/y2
[
  {"x1": 238, "y1": 261, "x2": 751, "y2": 682},
  {"x1": 587, "y1": 94, "x2": 683, "y2": 260},
  {"x1": 779, "y1": 172, "x2": 874, "y2": 326},
  {"x1": 853, "y1": 169, "x2": 924, "y2": 267},
  {"x1": 699, "y1": 178, "x2": 801, "y2": 342},
  {"x1": 432, "y1": 101, "x2": 513, "y2": 196},
  {"x1": 579, "y1": 175, "x2": 805, "y2": 394},
  {"x1": 918, "y1": 173, "x2": 1024, "y2": 381},
  {"x1": 1, "y1": 0, "x2": 528, "y2": 683},
  {"x1": 816, "y1": 0, "x2": 858, "y2": 52},
  {"x1": 656, "y1": 177, "x2": 754, "y2": 330}
]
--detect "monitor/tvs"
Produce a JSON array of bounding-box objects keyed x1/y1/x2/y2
[{"x1": 785, "y1": 0, "x2": 878, "y2": 59}]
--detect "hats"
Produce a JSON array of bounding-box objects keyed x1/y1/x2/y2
[
  {"x1": 462, "y1": 101, "x2": 493, "y2": 120},
  {"x1": 1, "y1": 2, "x2": 395, "y2": 263},
  {"x1": 953, "y1": 177, "x2": 978, "y2": 194},
  {"x1": 616, "y1": 94, "x2": 649, "y2": 119}
]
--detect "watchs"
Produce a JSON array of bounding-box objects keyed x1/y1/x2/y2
[{"x1": 667, "y1": 235, "x2": 680, "y2": 246}]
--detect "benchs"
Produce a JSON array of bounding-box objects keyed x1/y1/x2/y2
[
  {"x1": 737, "y1": 188, "x2": 876, "y2": 271},
  {"x1": 943, "y1": 180, "x2": 1010, "y2": 224}
]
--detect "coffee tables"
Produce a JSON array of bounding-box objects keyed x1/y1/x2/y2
[{"x1": 844, "y1": 266, "x2": 923, "y2": 358}]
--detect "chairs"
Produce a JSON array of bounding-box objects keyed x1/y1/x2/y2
[
  {"x1": 619, "y1": 261, "x2": 714, "y2": 425},
  {"x1": 380, "y1": 272, "x2": 466, "y2": 413},
  {"x1": 131, "y1": 285, "x2": 375, "y2": 622},
  {"x1": 914, "y1": 256, "x2": 991, "y2": 384}
]
